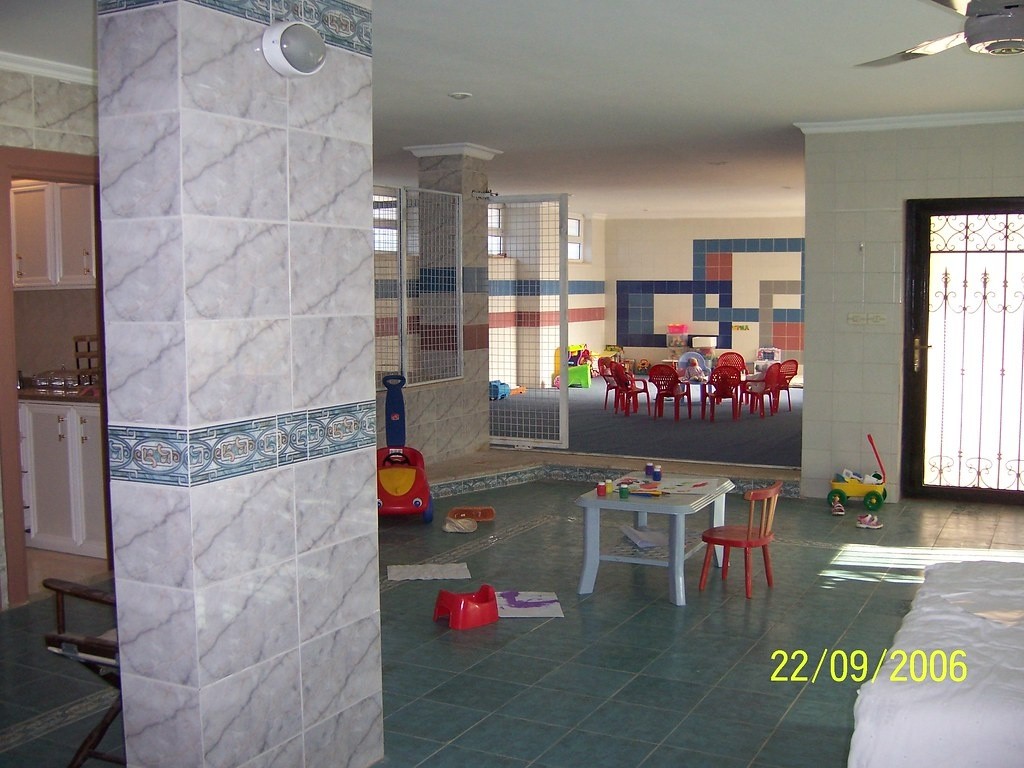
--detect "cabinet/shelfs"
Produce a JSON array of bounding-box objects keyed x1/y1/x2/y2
[
  {"x1": 16, "y1": 401, "x2": 107, "y2": 557},
  {"x1": 10, "y1": 177, "x2": 98, "y2": 289}
]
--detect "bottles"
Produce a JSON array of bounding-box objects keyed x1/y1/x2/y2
[
  {"x1": 619, "y1": 483, "x2": 629, "y2": 499},
  {"x1": 652, "y1": 465, "x2": 663, "y2": 481},
  {"x1": 645, "y1": 462, "x2": 654, "y2": 475},
  {"x1": 605, "y1": 479, "x2": 613, "y2": 494},
  {"x1": 597, "y1": 481, "x2": 606, "y2": 497}
]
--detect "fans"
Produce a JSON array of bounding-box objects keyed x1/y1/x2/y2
[{"x1": 853, "y1": 0, "x2": 1024, "y2": 75}]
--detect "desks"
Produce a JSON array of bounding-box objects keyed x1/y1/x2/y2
[
  {"x1": 575, "y1": 471, "x2": 736, "y2": 606},
  {"x1": 662, "y1": 359, "x2": 680, "y2": 370},
  {"x1": 753, "y1": 360, "x2": 783, "y2": 376}
]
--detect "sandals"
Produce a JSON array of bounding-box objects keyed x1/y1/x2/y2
[
  {"x1": 832, "y1": 503, "x2": 846, "y2": 516},
  {"x1": 856, "y1": 514, "x2": 884, "y2": 529}
]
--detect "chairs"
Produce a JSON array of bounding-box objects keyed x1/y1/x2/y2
[
  {"x1": 698, "y1": 480, "x2": 783, "y2": 598},
  {"x1": 599, "y1": 352, "x2": 799, "y2": 422}
]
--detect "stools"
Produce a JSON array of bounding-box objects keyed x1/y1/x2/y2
[{"x1": 39, "y1": 577, "x2": 127, "y2": 768}]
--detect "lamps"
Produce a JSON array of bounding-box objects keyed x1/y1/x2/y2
[{"x1": 259, "y1": 22, "x2": 326, "y2": 78}]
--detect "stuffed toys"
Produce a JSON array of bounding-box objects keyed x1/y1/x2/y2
[
  {"x1": 747, "y1": 361, "x2": 774, "y2": 391},
  {"x1": 686, "y1": 357, "x2": 706, "y2": 382}
]
